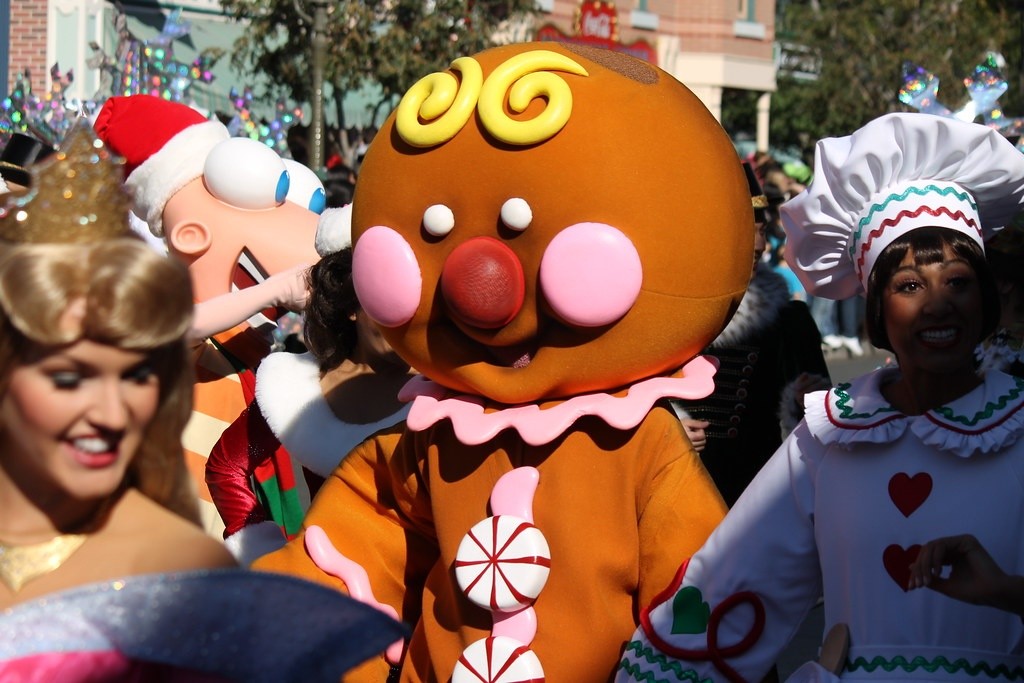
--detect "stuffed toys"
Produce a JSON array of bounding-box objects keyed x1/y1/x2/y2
[{"x1": 251, "y1": 41, "x2": 756, "y2": 683}]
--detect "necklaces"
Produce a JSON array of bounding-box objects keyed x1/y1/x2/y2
[{"x1": 0, "y1": 534, "x2": 87, "y2": 593}]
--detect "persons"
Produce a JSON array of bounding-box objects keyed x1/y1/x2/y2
[
  {"x1": 673, "y1": 149, "x2": 863, "y2": 506},
  {"x1": 204, "y1": 202, "x2": 414, "y2": 568},
  {"x1": 325, "y1": 165, "x2": 356, "y2": 207},
  {"x1": 94, "y1": 94, "x2": 326, "y2": 543},
  {"x1": 616, "y1": 112, "x2": 1024, "y2": 683},
  {"x1": 973, "y1": 229, "x2": 1023, "y2": 378},
  {"x1": 0, "y1": 229, "x2": 241, "y2": 683}
]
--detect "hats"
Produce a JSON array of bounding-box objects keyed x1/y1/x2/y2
[
  {"x1": 741, "y1": 162, "x2": 768, "y2": 223},
  {"x1": 775, "y1": 110, "x2": 1024, "y2": 300}
]
